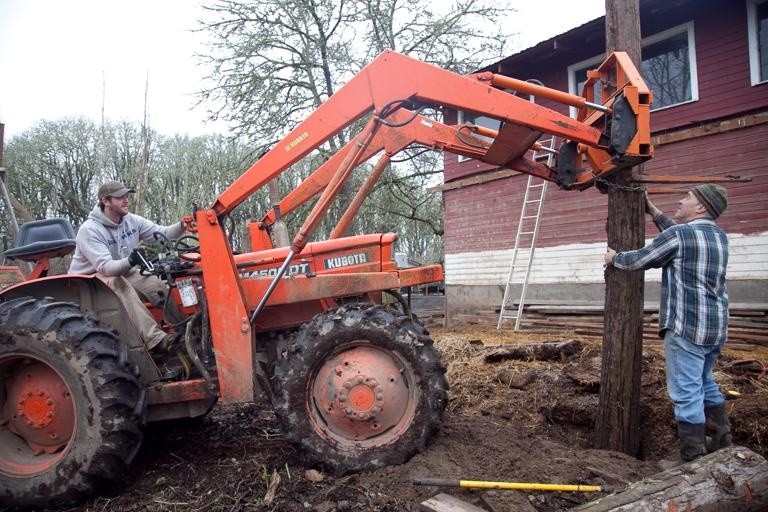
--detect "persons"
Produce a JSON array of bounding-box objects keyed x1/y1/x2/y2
[
  {"x1": 63, "y1": 177, "x2": 193, "y2": 356},
  {"x1": 604, "y1": 182, "x2": 733, "y2": 473}
]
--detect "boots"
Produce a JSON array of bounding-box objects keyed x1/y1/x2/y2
[
  {"x1": 658, "y1": 421, "x2": 709, "y2": 474},
  {"x1": 705, "y1": 402, "x2": 733, "y2": 450}
]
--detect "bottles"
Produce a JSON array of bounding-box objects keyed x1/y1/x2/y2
[{"x1": 272, "y1": 203, "x2": 290, "y2": 247}]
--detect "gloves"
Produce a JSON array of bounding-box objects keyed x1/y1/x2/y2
[{"x1": 127, "y1": 245, "x2": 149, "y2": 265}]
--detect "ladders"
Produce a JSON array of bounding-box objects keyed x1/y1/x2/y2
[{"x1": 496, "y1": 135, "x2": 557, "y2": 333}]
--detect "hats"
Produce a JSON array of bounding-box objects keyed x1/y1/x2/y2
[
  {"x1": 690, "y1": 184, "x2": 729, "y2": 221},
  {"x1": 97, "y1": 180, "x2": 136, "y2": 198}
]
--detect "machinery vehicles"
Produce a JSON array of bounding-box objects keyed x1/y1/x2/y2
[{"x1": 0, "y1": 46, "x2": 756, "y2": 512}]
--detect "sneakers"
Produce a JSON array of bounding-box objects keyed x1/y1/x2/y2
[{"x1": 154, "y1": 332, "x2": 183, "y2": 356}]
